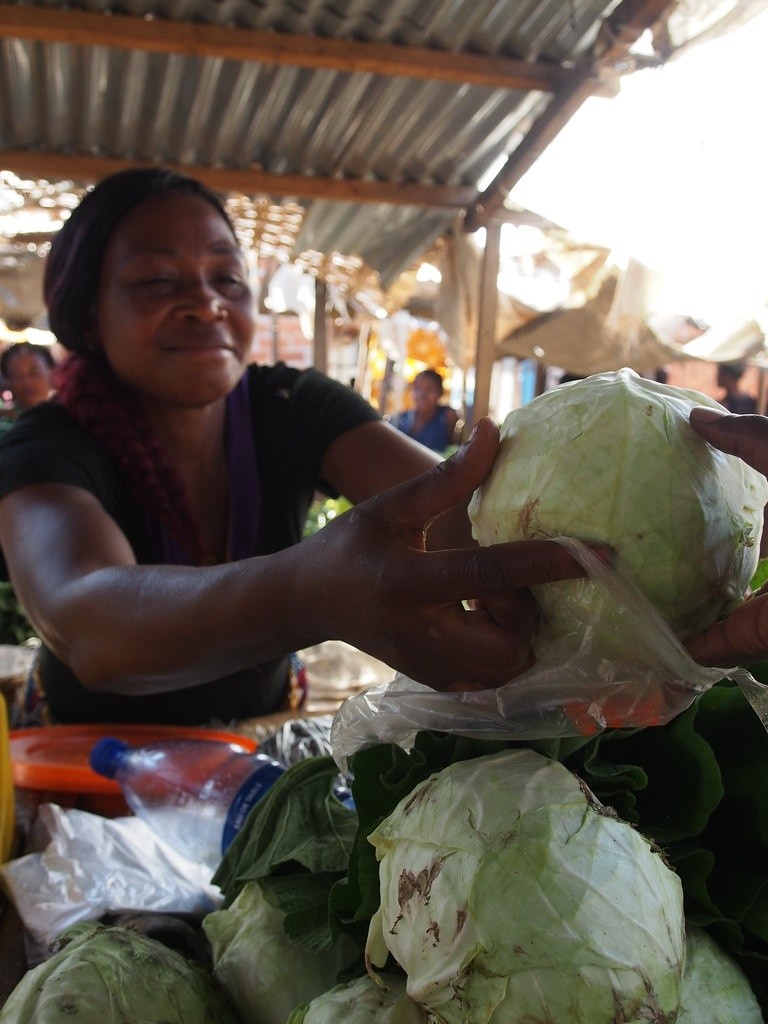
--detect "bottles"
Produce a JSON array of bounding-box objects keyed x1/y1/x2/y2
[{"x1": 89, "y1": 737, "x2": 353, "y2": 879}]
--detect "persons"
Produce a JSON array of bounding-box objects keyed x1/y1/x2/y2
[
  {"x1": 382, "y1": 370, "x2": 458, "y2": 451},
  {"x1": 678, "y1": 407, "x2": 768, "y2": 666},
  {"x1": 0, "y1": 167, "x2": 615, "y2": 730},
  {"x1": 0, "y1": 342, "x2": 57, "y2": 439},
  {"x1": 716, "y1": 361, "x2": 758, "y2": 415}
]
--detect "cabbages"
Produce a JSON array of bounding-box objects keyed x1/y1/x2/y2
[
  {"x1": 0, "y1": 749, "x2": 768, "y2": 1024},
  {"x1": 465, "y1": 364, "x2": 768, "y2": 654}
]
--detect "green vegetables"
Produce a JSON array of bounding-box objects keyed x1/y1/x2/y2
[{"x1": 211, "y1": 682, "x2": 768, "y2": 976}]
